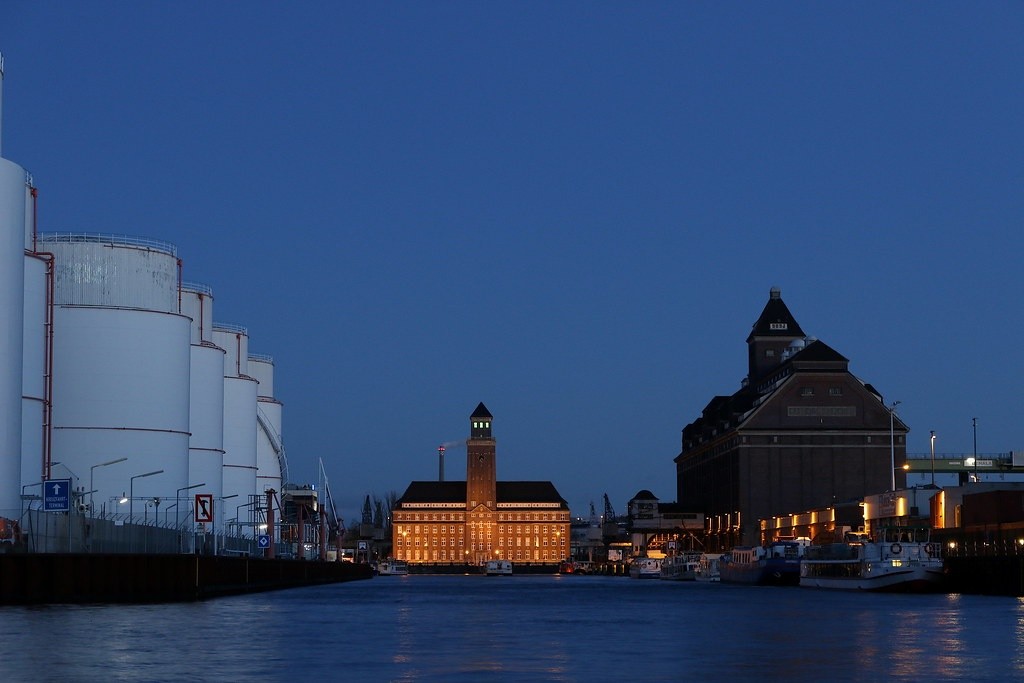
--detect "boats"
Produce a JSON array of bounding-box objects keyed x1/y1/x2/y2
[
  {"x1": 798, "y1": 540, "x2": 945, "y2": 595},
  {"x1": 486, "y1": 559, "x2": 513, "y2": 576},
  {"x1": 718, "y1": 541, "x2": 800, "y2": 588},
  {"x1": 629, "y1": 558, "x2": 661, "y2": 579},
  {"x1": 377, "y1": 560, "x2": 409, "y2": 576},
  {"x1": 659, "y1": 551, "x2": 721, "y2": 582},
  {"x1": 559, "y1": 563, "x2": 574, "y2": 574}
]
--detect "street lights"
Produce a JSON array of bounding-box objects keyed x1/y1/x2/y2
[
  {"x1": 20, "y1": 483, "x2": 42, "y2": 552},
  {"x1": 130, "y1": 469, "x2": 165, "y2": 554},
  {"x1": 930, "y1": 436, "x2": 937, "y2": 485},
  {"x1": 176, "y1": 483, "x2": 206, "y2": 553},
  {"x1": 69, "y1": 490, "x2": 99, "y2": 552},
  {"x1": 90, "y1": 456, "x2": 127, "y2": 551},
  {"x1": 402, "y1": 531, "x2": 406, "y2": 561},
  {"x1": 465, "y1": 551, "x2": 469, "y2": 565},
  {"x1": 891, "y1": 401, "x2": 910, "y2": 492},
  {"x1": 237, "y1": 501, "x2": 260, "y2": 556},
  {"x1": 495, "y1": 550, "x2": 499, "y2": 560},
  {"x1": 973, "y1": 417, "x2": 979, "y2": 483},
  {"x1": 212, "y1": 494, "x2": 239, "y2": 556}
]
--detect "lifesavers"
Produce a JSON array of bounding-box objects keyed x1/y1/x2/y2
[
  {"x1": 641, "y1": 564, "x2": 647, "y2": 568},
  {"x1": 890, "y1": 543, "x2": 902, "y2": 555},
  {"x1": 924, "y1": 543, "x2": 934, "y2": 554}
]
